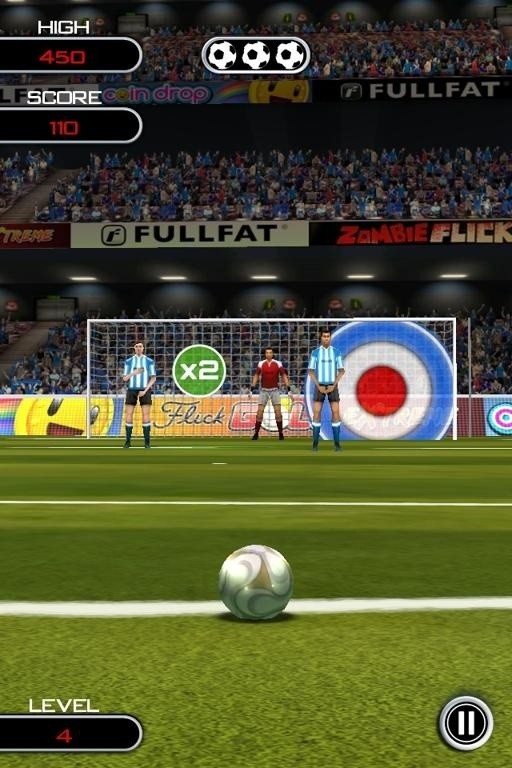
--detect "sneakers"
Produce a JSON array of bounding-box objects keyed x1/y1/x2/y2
[
  {"x1": 313, "y1": 447, "x2": 317, "y2": 451},
  {"x1": 279, "y1": 437, "x2": 284, "y2": 440},
  {"x1": 335, "y1": 447, "x2": 344, "y2": 452},
  {"x1": 145, "y1": 444, "x2": 150, "y2": 448},
  {"x1": 252, "y1": 436, "x2": 258, "y2": 440},
  {"x1": 125, "y1": 445, "x2": 130, "y2": 448}
]
